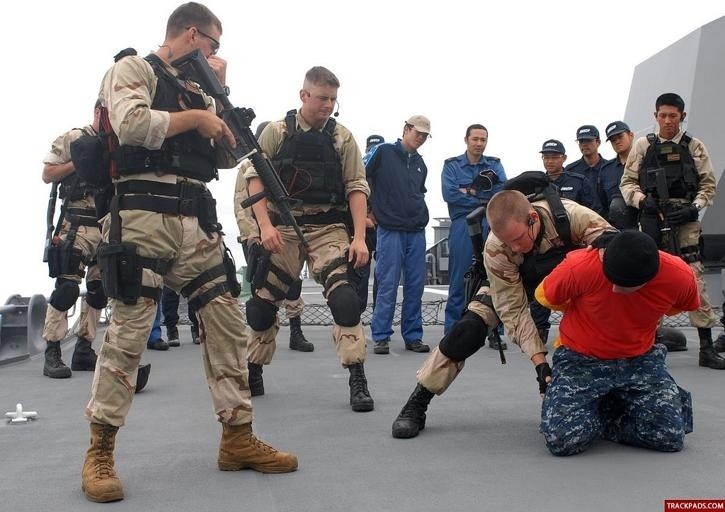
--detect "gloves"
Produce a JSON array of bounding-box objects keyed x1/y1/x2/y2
[
  {"x1": 536, "y1": 364, "x2": 553, "y2": 393},
  {"x1": 590, "y1": 232, "x2": 617, "y2": 248},
  {"x1": 642, "y1": 197, "x2": 661, "y2": 216},
  {"x1": 667, "y1": 205, "x2": 699, "y2": 225}
]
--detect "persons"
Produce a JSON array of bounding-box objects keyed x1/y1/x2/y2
[
  {"x1": 234, "y1": 121, "x2": 314, "y2": 352},
  {"x1": 534, "y1": 231, "x2": 700, "y2": 457},
  {"x1": 362, "y1": 115, "x2": 432, "y2": 353},
  {"x1": 44, "y1": 101, "x2": 102, "y2": 378},
  {"x1": 145, "y1": 303, "x2": 170, "y2": 352},
  {"x1": 393, "y1": 189, "x2": 614, "y2": 438},
  {"x1": 81, "y1": 3, "x2": 298, "y2": 502},
  {"x1": 566, "y1": 124, "x2": 610, "y2": 188},
  {"x1": 361, "y1": 134, "x2": 386, "y2": 314},
  {"x1": 539, "y1": 141, "x2": 598, "y2": 216},
  {"x1": 243, "y1": 66, "x2": 375, "y2": 411},
  {"x1": 599, "y1": 120, "x2": 641, "y2": 229},
  {"x1": 162, "y1": 285, "x2": 200, "y2": 346},
  {"x1": 442, "y1": 125, "x2": 509, "y2": 349},
  {"x1": 619, "y1": 93, "x2": 725, "y2": 370}
]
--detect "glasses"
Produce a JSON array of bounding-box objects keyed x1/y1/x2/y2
[{"x1": 185, "y1": 26, "x2": 220, "y2": 55}]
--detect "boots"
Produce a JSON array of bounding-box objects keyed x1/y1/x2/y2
[
  {"x1": 82, "y1": 423, "x2": 124, "y2": 502},
  {"x1": 71, "y1": 338, "x2": 98, "y2": 371},
  {"x1": 248, "y1": 362, "x2": 264, "y2": 396},
  {"x1": 697, "y1": 327, "x2": 724, "y2": 369},
  {"x1": 168, "y1": 328, "x2": 180, "y2": 346},
  {"x1": 349, "y1": 364, "x2": 374, "y2": 411},
  {"x1": 712, "y1": 334, "x2": 724, "y2": 351},
  {"x1": 191, "y1": 326, "x2": 200, "y2": 344},
  {"x1": 218, "y1": 423, "x2": 298, "y2": 473},
  {"x1": 289, "y1": 318, "x2": 314, "y2": 352},
  {"x1": 392, "y1": 383, "x2": 435, "y2": 438},
  {"x1": 43, "y1": 341, "x2": 72, "y2": 378}
]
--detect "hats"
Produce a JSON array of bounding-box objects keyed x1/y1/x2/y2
[
  {"x1": 602, "y1": 231, "x2": 659, "y2": 288},
  {"x1": 539, "y1": 140, "x2": 565, "y2": 154},
  {"x1": 605, "y1": 121, "x2": 630, "y2": 142},
  {"x1": 575, "y1": 126, "x2": 599, "y2": 140},
  {"x1": 367, "y1": 136, "x2": 383, "y2": 151},
  {"x1": 406, "y1": 115, "x2": 432, "y2": 138}
]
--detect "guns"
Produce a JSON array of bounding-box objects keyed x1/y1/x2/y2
[
  {"x1": 246, "y1": 243, "x2": 259, "y2": 282},
  {"x1": 53, "y1": 236, "x2": 72, "y2": 273},
  {"x1": 101, "y1": 242, "x2": 116, "y2": 297},
  {"x1": 645, "y1": 134, "x2": 679, "y2": 256},
  {"x1": 467, "y1": 206, "x2": 506, "y2": 363},
  {"x1": 171, "y1": 48, "x2": 312, "y2": 252},
  {"x1": 42, "y1": 182, "x2": 59, "y2": 262}
]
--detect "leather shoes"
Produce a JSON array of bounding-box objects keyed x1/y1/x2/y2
[
  {"x1": 147, "y1": 339, "x2": 169, "y2": 351},
  {"x1": 374, "y1": 340, "x2": 390, "y2": 353},
  {"x1": 406, "y1": 342, "x2": 430, "y2": 352},
  {"x1": 489, "y1": 335, "x2": 506, "y2": 349}
]
